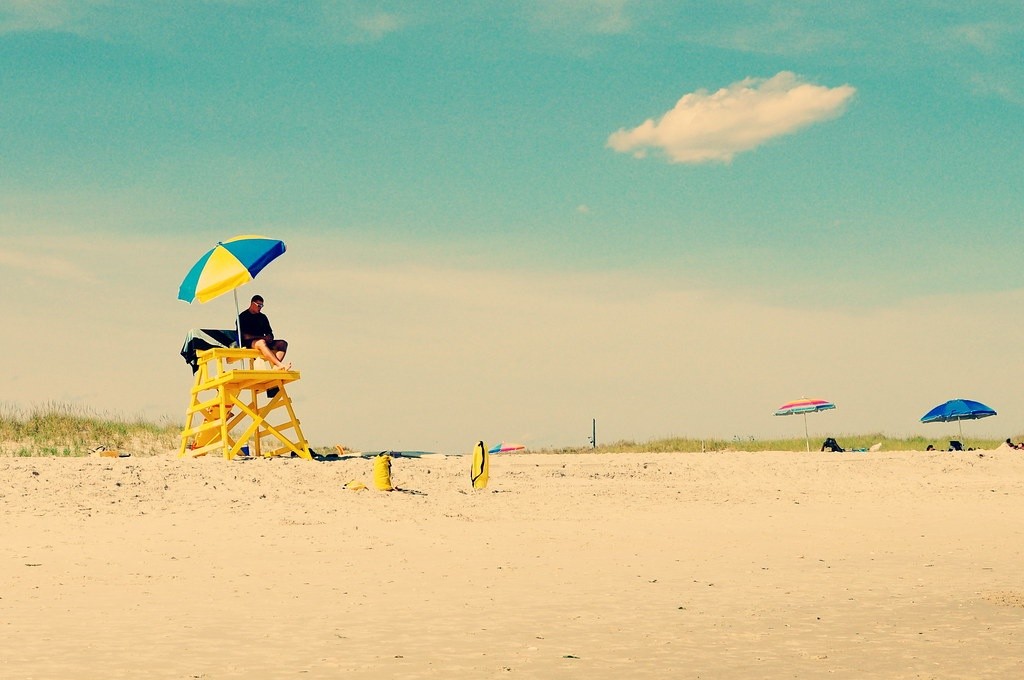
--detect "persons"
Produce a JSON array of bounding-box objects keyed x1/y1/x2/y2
[
  {"x1": 235, "y1": 295, "x2": 291, "y2": 371},
  {"x1": 927, "y1": 445, "x2": 933, "y2": 451},
  {"x1": 1006, "y1": 439, "x2": 1014, "y2": 447}
]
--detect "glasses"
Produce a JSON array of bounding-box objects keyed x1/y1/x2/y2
[{"x1": 253, "y1": 302, "x2": 263, "y2": 308}]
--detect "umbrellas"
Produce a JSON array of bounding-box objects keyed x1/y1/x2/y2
[
  {"x1": 177, "y1": 234, "x2": 286, "y2": 348},
  {"x1": 774, "y1": 399, "x2": 835, "y2": 451},
  {"x1": 488, "y1": 443, "x2": 525, "y2": 453},
  {"x1": 919, "y1": 399, "x2": 997, "y2": 442}
]
